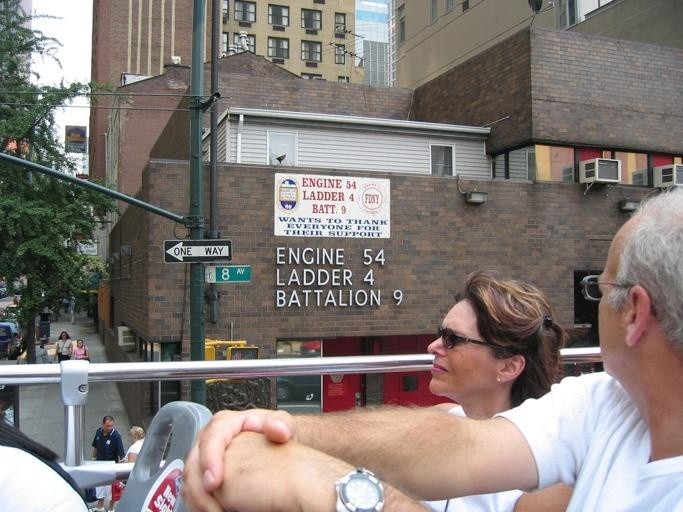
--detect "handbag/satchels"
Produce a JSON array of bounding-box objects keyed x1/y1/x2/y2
[
  {"x1": 85, "y1": 488, "x2": 97, "y2": 503},
  {"x1": 112, "y1": 480, "x2": 125, "y2": 501}
]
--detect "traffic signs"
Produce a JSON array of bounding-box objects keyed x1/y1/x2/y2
[
  {"x1": 207, "y1": 266, "x2": 252, "y2": 282},
  {"x1": 164, "y1": 237, "x2": 232, "y2": 264}
]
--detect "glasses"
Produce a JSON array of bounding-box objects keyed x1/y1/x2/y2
[
  {"x1": 438, "y1": 326, "x2": 487, "y2": 349},
  {"x1": 580, "y1": 275, "x2": 622, "y2": 302}
]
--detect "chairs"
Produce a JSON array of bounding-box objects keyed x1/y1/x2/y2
[{"x1": 110, "y1": 400, "x2": 212, "y2": 511}]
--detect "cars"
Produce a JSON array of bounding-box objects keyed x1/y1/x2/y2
[
  {"x1": 0, "y1": 281, "x2": 71, "y2": 360},
  {"x1": 275, "y1": 350, "x2": 320, "y2": 402}
]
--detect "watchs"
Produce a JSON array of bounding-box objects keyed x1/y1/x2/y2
[{"x1": 331, "y1": 466, "x2": 386, "y2": 512}]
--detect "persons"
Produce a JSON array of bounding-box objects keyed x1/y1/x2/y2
[
  {"x1": 120, "y1": 425, "x2": 147, "y2": 462},
  {"x1": 417, "y1": 269, "x2": 570, "y2": 512},
  {"x1": 13, "y1": 296, "x2": 18, "y2": 304},
  {"x1": 0, "y1": 412, "x2": 92, "y2": 511},
  {"x1": 177, "y1": 183, "x2": 683, "y2": 512},
  {"x1": 63, "y1": 298, "x2": 69, "y2": 313},
  {"x1": 53, "y1": 330, "x2": 73, "y2": 363},
  {"x1": 73, "y1": 339, "x2": 90, "y2": 362},
  {"x1": 69, "y1": 299, "x2": 77, "y2": 325},
  {"x1": 89, "y1": 415, "x2": 125, "y2": 512}
]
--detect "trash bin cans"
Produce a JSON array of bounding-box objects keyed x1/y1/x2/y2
[{"x1": 38, "y1": 312, "x2": 50, "y2": 338}]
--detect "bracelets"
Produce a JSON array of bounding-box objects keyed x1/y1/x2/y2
[{"x1": 91, "y1": 456, "x2": 97, "y2": 460}]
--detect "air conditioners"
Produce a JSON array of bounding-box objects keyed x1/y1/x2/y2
[
  {"x1": 579, "y1": 156, "x2": 682, "y2": 197},
  {"x1": 116, "y1": 326, "x2": 134, "y2": 346}
]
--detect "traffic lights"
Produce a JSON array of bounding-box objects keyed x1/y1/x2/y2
[{"x1": 225, "y1": 346, "x2": 259, "y2": 362}]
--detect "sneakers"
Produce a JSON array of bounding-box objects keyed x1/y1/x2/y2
[{"x1": 89, "y1": 507, "x2": 115, "y2": 512}]
